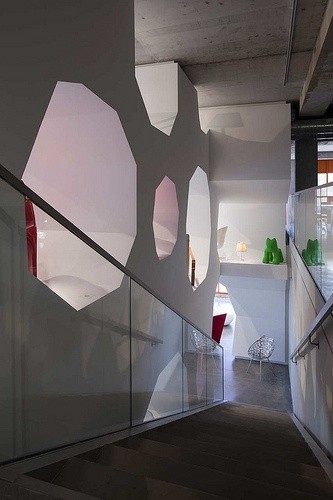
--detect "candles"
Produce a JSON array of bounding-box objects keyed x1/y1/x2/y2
[{"x1": 236, "y1": 241, "x2": 246, "y2": 252}]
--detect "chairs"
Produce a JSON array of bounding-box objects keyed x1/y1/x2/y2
[
  {"x1": 189, "y1": 330, "x2": 216, "y2": 371},
  {"x1": 243, "y1": 335, "x2": 278, "y2": 381}
]
